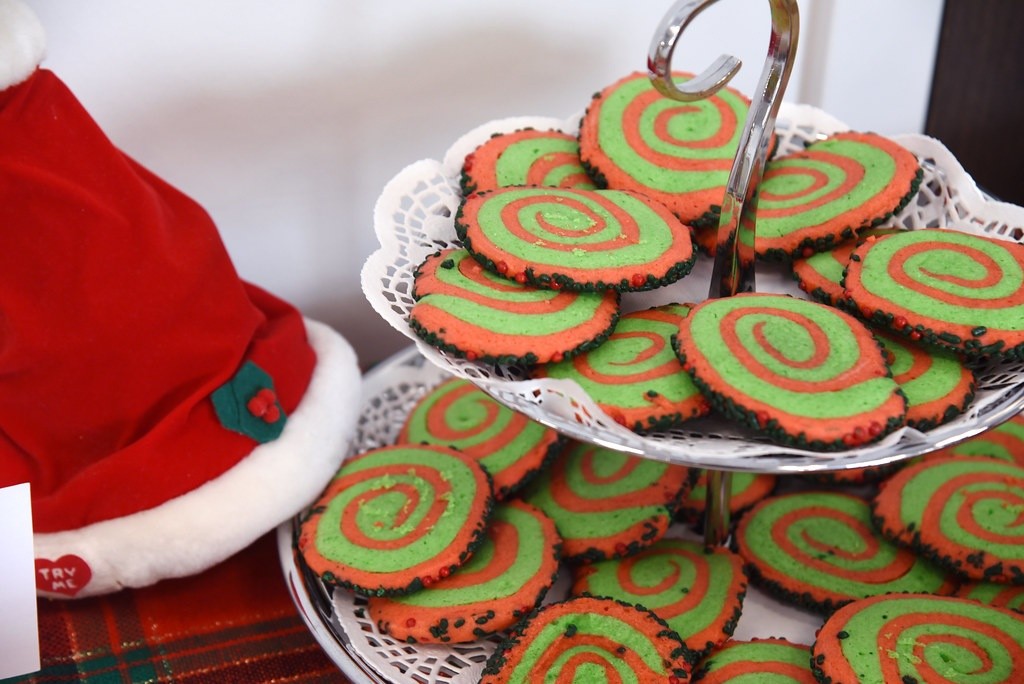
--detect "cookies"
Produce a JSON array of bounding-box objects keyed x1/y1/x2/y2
[{"x1": 299, "y1": 66, "x2": 1024, "y2": 684}]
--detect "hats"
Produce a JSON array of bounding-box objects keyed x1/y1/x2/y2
[{"x1": 0, "y1": 0, "x2": 363, "y2": 600}]
